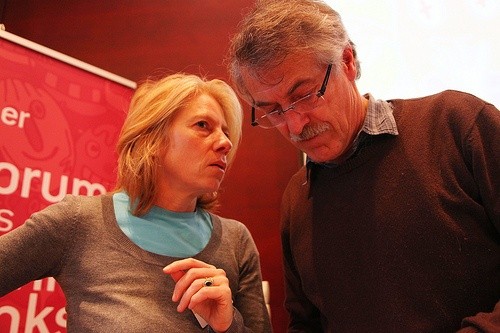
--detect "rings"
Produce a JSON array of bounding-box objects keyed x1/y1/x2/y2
[{"x1": 203, "y1": 276, "x2": 213, "y2": 287}]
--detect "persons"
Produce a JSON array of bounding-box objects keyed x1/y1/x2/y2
[
  {"x1": 225, "y1": 0, "x2": 500, "y2": 333},
  {"x1": 0, "y1": 73, "x2": 273, "y2": 333}
]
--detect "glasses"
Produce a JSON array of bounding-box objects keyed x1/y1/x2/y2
[{"x1": 251, "y1": 64, "x2": 332, "y2": 129}]
